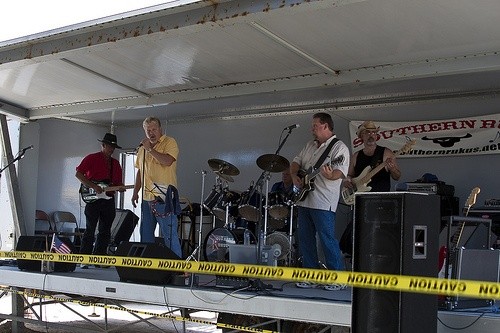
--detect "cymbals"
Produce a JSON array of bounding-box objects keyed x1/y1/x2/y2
[
  {"x1": 256, "y1": 154, "x2": 290, "y2": 172},
  {"x1": 208, "y1": 159, "x2": 240, "y2": 176}
]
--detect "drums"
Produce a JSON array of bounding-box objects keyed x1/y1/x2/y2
[
  {"x1": 268, "y1": 192, "x2": 289, "y2": 217},
  {"x1": 203, "y1": 227, "x2": 258, "y2": 264},
  {"x1": 211, "y1": 190, "x2": 242, "y2": 223},
  {"x1": 238, "y1": 191, "x2": 265, "y2": 222},
  {"x1": 202, "y1": 188, "x2": 222, "y2": 214}
]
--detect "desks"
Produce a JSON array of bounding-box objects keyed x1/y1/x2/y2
[{"x1": 439, "y1": 216, "x2": 492, "y2": 308}]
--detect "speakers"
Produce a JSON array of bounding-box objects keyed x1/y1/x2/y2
[
  {"x1": 109, "y1": 209, "x2": 183, "y2": 286},
  {"x1": 16, "y1": 235, "x2": 77, "y2": 272},
  {"x1": 352, "y1": 192, "x2": 440, "y2": 333}
]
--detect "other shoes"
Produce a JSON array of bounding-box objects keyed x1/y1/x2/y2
[
  {"x1": 94, "y1": 264, "x2": 110, "y2": 268},
  {"x1": 80, "y1": 264, "x2": 88, "y2": 269}
]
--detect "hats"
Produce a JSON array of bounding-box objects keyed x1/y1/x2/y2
[
  {"x1": 357, "y1": 121, "x2": 379, "y2": 137},
  {"x1": 97, "y1": 133, "x2": 121, "y2": 149}
]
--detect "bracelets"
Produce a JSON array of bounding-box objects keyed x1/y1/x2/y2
[{"x1": 148, "y1": 148, "x2": 154, "y2": 153}]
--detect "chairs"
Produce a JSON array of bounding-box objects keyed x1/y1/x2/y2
[
  {"x1": 53, "y1": 211, "x2": 78, "y2": 237},
  {"x1": 35, "y1": 210, "x2": 58, "y2": 236}
]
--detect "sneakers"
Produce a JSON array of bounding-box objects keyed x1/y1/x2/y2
[
  {"x1": 295, "y1": 282, "x2": 319, "y2": 288},
  {"x1": 323, "y1": 284, "x2": 345, "y2": 291}
]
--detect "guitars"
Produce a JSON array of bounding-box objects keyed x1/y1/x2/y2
[
  {"x1": 437, "y1": 185, "x2": 482, "y2": 302},
  {"x1": 80, "y1": 179, "x2": 135, "y2": 204},
  {"x1": 293, "y1": 154, "x2": 346, "y2": 202},
  {"x1": 342, "y1": 139, "x2": 418, "y2": 205}
]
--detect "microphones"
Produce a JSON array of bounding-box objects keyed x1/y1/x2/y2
[
  {"x1": 284, "y1": 124, "x2": 300, "y2": 130},
  {"x1": 19, "y1": 144, "x2": 34, "y2": 152},
  {"x1": 139, "y1": 143, "x2": 143, "y2": 147}
]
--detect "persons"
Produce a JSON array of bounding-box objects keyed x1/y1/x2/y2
[
  {"x1": 270, "y1": 165, "x2": 294, "y2": 200},
  {"x1": 290, "y1": 113, "x2": 350, "y2": 291},
  {"x1": 75, "y1": 133, "x2": 126, "y2": 269},
  {"x1": 339, "y1": 120, "x2": 402, "y2": 258},
  {"x1": 131, "y1": 116, "x2": 183, "y2": 276}
]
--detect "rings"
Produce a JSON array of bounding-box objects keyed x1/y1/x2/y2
[{"x1": 389, "y1": 162, "x2": 391, "y2": 164}]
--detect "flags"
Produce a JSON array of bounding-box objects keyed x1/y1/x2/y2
[{"x1": 52, "y1": 235, "x2": 71, "y2": 255}]
